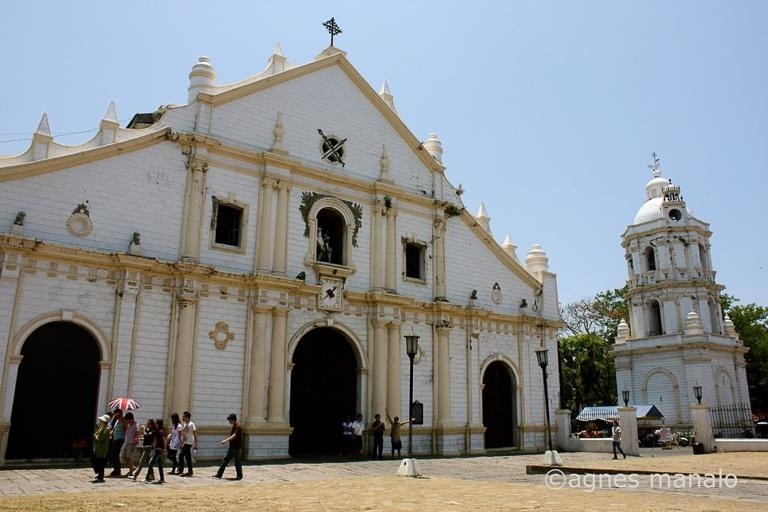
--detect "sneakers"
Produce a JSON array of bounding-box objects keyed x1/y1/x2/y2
[
  {"x1": 236, "y1": 475, "x2": 243, "y2": 480},
  {"x1": 624, "y1": 454, "x2": 626, "y2": 459},
  {"x1": 612, "y1": 458, "x2": 617, "y2": 459},
  {"x1": 93, "y1": 468, "x2": 193, "y2": 483},
  {"x1": 212, "y1": 475, "x2": 221, "y2": 479}
]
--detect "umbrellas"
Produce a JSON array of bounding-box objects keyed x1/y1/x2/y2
[{"x1": 105, "y1": 397, "x2": 144, "y2": 412}]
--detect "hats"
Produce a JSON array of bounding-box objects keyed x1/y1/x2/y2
[{"x1": 97, "y1": 414, "x2": 111, "y2": 423}]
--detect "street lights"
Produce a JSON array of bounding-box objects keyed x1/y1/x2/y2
[
  {"x1": 397, "y1": 320, "x2": 425, "y2": 479},
  {"x1": 619, "y1": 385, "x2": 630, "y2": 408},
  {"x1": 691, "y1": 379, "x2": 704, "y2": 404},
  {"x1": 534, "y1": 343, "x2": 564, "y2": 465}
]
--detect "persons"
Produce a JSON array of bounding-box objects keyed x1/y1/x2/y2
[
  {"x1": 211, "y1": 413, "x2": 243, "y2": 480},
  {"x1": 352, "y1": 413, "x2": 366, "y2": 458},
  {"x1": 611, "y1": 421, "x2": 626, "y2": 460},
  {"x1": 342, "y1": 418, "x2": 352, "y2": 460},
  {"x1": 372, "y1": 414, "x2": 385, "y2": 460},
  {"x1": 385, "y1": 407, "x2": 416, "y2": 459},
  {"x1": 90, "y1": 409, "x2": 200, "y2": 484}
]
